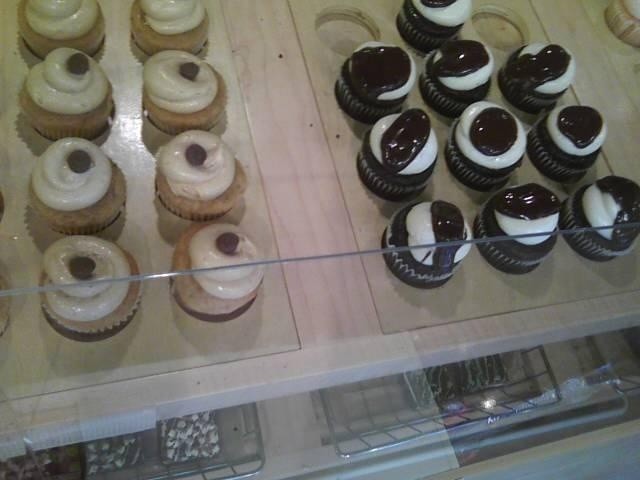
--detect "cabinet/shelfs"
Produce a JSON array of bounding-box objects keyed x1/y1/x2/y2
[{"x1": 0, "y1": 0, "x2": 640, "y2": 480}]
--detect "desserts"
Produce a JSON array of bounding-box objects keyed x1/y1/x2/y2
[
  {"x1": 332, "y1": 2, "x2": 639, "y2": 290},
  {"x1": 14, "y1": 0, "x2": 269, "y2": 335}
]
